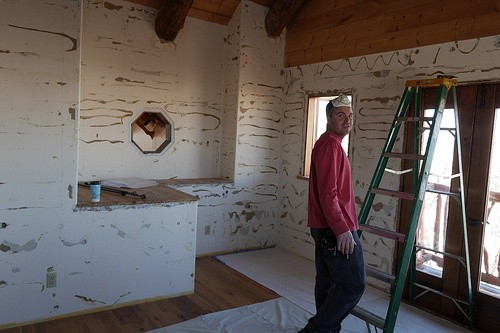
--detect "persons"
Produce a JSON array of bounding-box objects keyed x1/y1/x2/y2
[{"x1": 297, "y1": 95, "x2": 368, "y2": 333}]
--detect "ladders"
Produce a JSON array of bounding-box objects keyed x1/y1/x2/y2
[{"x1": 325, "y1": 75, "x2": 479, "y2": 332}]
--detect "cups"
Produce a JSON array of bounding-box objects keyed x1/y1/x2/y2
[{"x1": 90, "y1": 181, "x2": 101, "y2": 202}]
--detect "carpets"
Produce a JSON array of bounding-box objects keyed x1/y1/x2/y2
[{"x1": 144, "y1": 245, "x2": 472, "y2": 332}]
